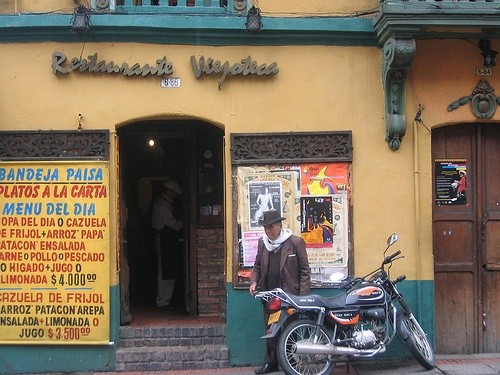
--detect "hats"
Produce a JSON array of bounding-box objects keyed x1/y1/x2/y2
[
  {"x1": 459, "y1": 170, "x2": 466, "y2": 177},
  {"x1": 259, "y1": 211, "x2": 286, "y2": 227},
  {"x1": 164, "y1": 179, "x2": 183, "y2": 194}
]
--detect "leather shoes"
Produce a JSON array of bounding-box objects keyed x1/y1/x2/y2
[{"x1": 255, "y1": 363, "x2": 279, "y2": 374}]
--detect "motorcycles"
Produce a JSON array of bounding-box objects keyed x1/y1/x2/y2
[{"x1": 255, "y1": 233, "x2": 435, "y2": 375}]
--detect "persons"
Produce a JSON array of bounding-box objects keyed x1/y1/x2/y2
[
  {"x1": 250, "y1": 210, "x2": 310, "y2": 375},
  {"x1": 151, "y1": 180, "x2": 185, "y2": 312},
  {"x1": 453, "y1": 170, "x2": 467, "y2": 197},
  {"x1": 252, "y1": 186, "x2": 278, "y2": 224}
]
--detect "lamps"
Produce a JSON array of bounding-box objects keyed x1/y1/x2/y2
[
  {"x1": 479, "y1": 38, "x2": 500, "y2": 68},
  {"x1": 71, "y1": 5, "x2": 91, "y2": 34},
  {"x1": 245, "y1": 5, "x2": 263, "y2": 33}
]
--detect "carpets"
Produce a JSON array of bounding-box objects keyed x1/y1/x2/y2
[{"x1": 129, "y1": 314, "x2": 225, "y2": 326}]
